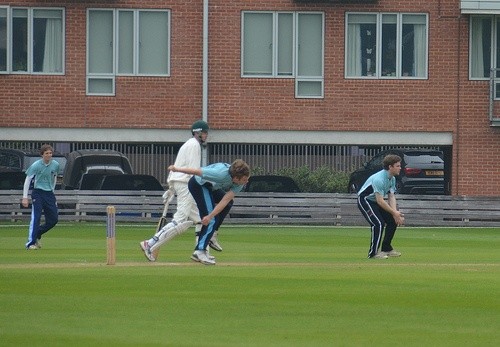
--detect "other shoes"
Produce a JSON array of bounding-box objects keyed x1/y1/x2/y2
[
  {"x1": 35, "y1": 239, "x2": 41, "y2": 248},
  {"x1": 29, "y1": 245, "x2": 39, "y2": 250}
]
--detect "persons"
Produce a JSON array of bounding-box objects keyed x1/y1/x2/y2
[
  {"x1": 167, "y1": 158, "x2": 251, "y2": 266},
  {"x1": 357, "y1": 155, "x2": 405, "y2": 259},
  {"x1": 22, "y1": 144, "x2": 60, "y2": 250},
  {"x1": 139, "y1": 120, "x2": 223, "y2": 262}
]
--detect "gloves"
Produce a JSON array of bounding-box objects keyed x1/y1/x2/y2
[{"x1": 163, "y1": 189, "x2": 175, "y2": 205}]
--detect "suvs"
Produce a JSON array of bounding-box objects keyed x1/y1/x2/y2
[
  {"x1": 245, "y1": 175, "x2": 311, "y2": 218},
  {"x1": 0, "y1": 148, "x2": 32, "y2": 215},
  {"x1": 348, "y1": 148, "x2": 445, "y2": 196},
  {"x1": 61, "y1": 148, "x2": 165, "y2": 218}
]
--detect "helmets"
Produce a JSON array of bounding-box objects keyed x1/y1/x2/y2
[{"x1": 192, "y1": 120, "x2": 209, "y2": 134}]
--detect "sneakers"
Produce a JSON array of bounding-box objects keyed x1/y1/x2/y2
[
  {"x1": 373, "y1": 254, "x2": 388, "y2": 259},
  {"x1": 189, "y1": 250, "x2": 215, "y2": 265},
  {"x1": 380, "y1": 250, "x2": 401, "y2": 257},
  {"x1": 140, "y1": 239, "x2": 155, "y2": 262},
  {"x1": 209, "y1": 239, "x2": 222, "y2": 252}
]
